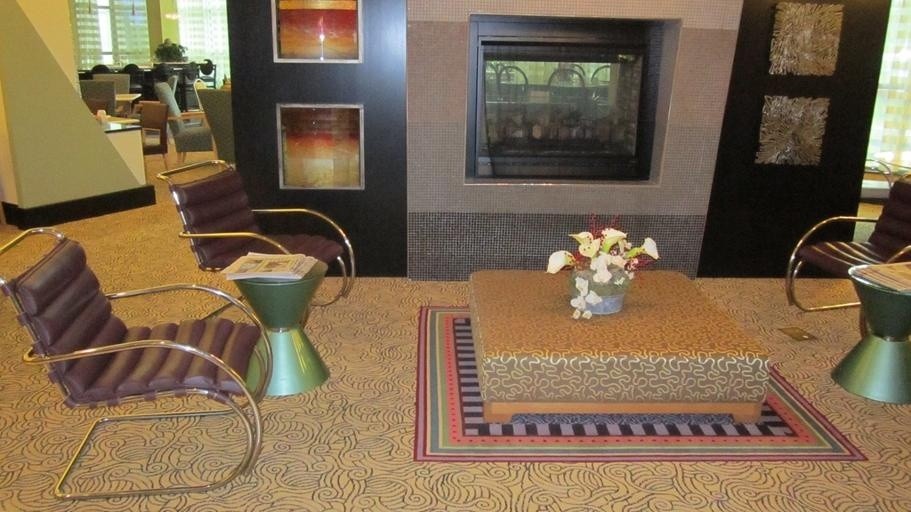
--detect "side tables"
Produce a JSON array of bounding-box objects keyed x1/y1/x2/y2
[
  {"x1": 831, "y1": 262, "x2": 909, "y2": 408},
  {"x1": 231, "y1": 258, "x2": 331, "y2": 398}
]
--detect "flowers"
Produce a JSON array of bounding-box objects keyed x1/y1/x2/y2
[{"x1": 549, "y1": 222, "x2": 660, "y2": 321}]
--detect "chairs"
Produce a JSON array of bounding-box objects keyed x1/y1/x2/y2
[
  {"x1": 92, "y1": 73, "x2": 130, "y2": 94},
  {"x1": 0, "y1": 227, "x2": 275, "y2": 488},
  {"x1": 80, "y1": 79, "x2": 115, "y2": 118},
  {"x1": 197, "y1": 85, "x2": 235, "y2": 160},
  {"x1": 167, "y1": 75, "x2": 178, "y2": 94},
  {"x1": 157, "y1": 160, "x2": 356, "y2": 308},
  {"x1": 192, "y1": 78, "x2": 207, "y2": 111},
  {"x1": 786, "y1": 171, "x2": 910, "y2": 313},
  {"x1": 153, "y1": 81, "x2": 212, "y2": 163},
  {"x1": 140, "y1": 99, "x2": 170, "y2": 176}
]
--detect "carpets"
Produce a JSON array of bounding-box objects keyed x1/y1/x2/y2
[{"x1": 411, "y1": 306, "x2": 869, "y2": 463}]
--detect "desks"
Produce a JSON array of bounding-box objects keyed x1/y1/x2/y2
[
  {"x1": 468, "y1": 267, "x2": 770, "y2": 425},
  {"x1": 116, "y1": 93, "x2": 141, "y2": 114}
]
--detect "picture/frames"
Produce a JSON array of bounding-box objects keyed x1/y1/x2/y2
[
  {"x1": 275, "y1": 101, "x2": 365, "y2": 191},
  {"x1": 270, "y1": 0, "x2": 364, "y2": 64}
]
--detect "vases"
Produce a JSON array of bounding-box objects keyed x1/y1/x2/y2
[{"x1": 564, "y1": 268, "x2": 625, "y2": 316}]
